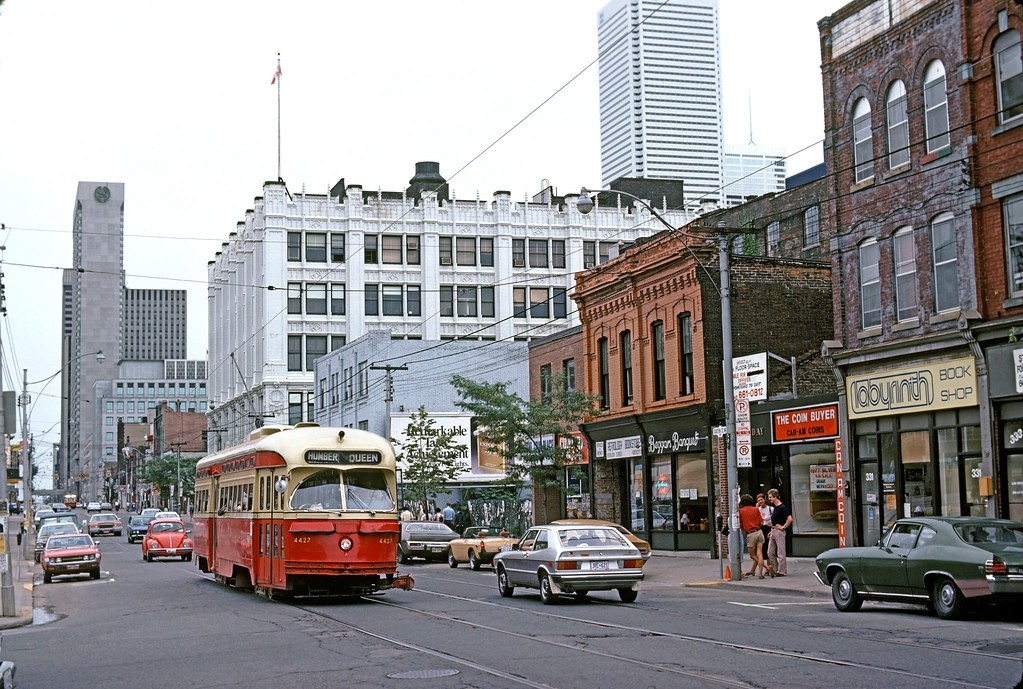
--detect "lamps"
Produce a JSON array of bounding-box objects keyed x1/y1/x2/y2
[{"x1": 979, "y1": 475, "x2": 993, "y2": 498}]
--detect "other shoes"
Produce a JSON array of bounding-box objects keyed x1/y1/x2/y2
[
  {"x1": 745, "y1": 572, "x2": 755, "y2": 576},
  {"x1": 759, "y1": 576, "x2": 764, "y2": 579},
  {"x1": 775, "y1": 572, "x2": 783, "y2": 577},
  {"x1": 768, "y1": 566, "x2": 774, "y2": 578},
  {"x1": 764, "y1": 571, "x2": 768, "y2": 576}
]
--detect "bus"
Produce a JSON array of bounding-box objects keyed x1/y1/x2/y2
[
  {"x1": 63, "y1": 495, "x2": 77, "y2": 509},
  {"x1": 191, "y1": 422, "x2": 415, "y2": 605}
]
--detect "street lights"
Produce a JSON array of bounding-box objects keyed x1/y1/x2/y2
[
  {"x1": 396, "y1": 468, "x2": 404, "y2": 510},
  {"x1": 29, "y1": 419, "x2": 74, "y2": 501},
  {"x1": 574, "y1": 186, "x2": 742, "y2": 583},
  {"x1": 22, "y1": 350, "x2": 106, "y2": 562}
]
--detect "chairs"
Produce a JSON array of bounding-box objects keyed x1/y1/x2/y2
[
  {"x1": 477, "y1": 531, "x2": 508, "y2": 537},
  {"x1": 567, "y1": 534, "x2": 601, "y2": 547}
]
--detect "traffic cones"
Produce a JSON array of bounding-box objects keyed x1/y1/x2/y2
[{"x1": 723, "y1": 564, "x2": 731, "y2": 582}]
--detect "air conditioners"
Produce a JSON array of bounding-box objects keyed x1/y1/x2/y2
[
  {"x1": 586, "y1": 263, "x2": 593, "y2": 269},
  {"x1": 407, "y1": 242, "x2": 418, "y2": 250},
  {"x1": 513, "y1": 260, "x2": 524, "y2": 266},
  {"x1": 440, "y1": 257, "x2": 451, "y2": 265}
]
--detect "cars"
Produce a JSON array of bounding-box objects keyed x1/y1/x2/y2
[
  {"x1": 40, "y1": 534, "x2": 101, "y2": 584},
  {"x1": 76, "y1": 501, "x2": 112, "y2": 513},
  {"x1": 127, "y1": 508, "x2": 183, "y2": 545},
  {"x1": 141, "y1": 519, "x2": 193, "y2": 563},
  {"x1": 631, "y1": 503, "x2": 673, "y2": 530},
  {"x1": 88, "y1": 514, "x2": 123, "y2": 537},
  {"x1": 13, "y1": 500, "x2": 83, "y2": 561},
  {"x1": 521, "y1": 518, "x2": 652, "y2": 565},
  {"x1": 492, "y1": 524, "x2": 645, "y2": 606},
  {"x1": 397, "y1": 521, "x2": 461, "y2": 565},
  {"x1": 812, "y1": 516, "x2": 1023, "y2": 622},
  {"x1": 447, "y1": 526, "x2": 522, "y2": 572}
]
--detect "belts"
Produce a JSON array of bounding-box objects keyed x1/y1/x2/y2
[{"x1": 746, "y1": 528, "x2": 760, "y2": 534}]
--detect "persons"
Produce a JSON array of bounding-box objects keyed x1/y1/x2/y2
[
  {"x1": 766, "y1": 489, "x2": 794, "y2": 576},
  {"x1": 746, "y1": 493, "x2": 774, "y2": 576},
  {"x1": 16, "y1": 501, "x2": 20, "y2": 515},
  {"x1": 681, "y1": 508, "x2": 691, "y2": 525},
  {"x1": 442, "y1": 503, "x2": 455, "y2": 528},
  {"x1": 357, "y1": 475, "x2": 383, "y2": 499},
  {"x1": 218, "y1": 503, "x2": 227, "y2": 516},
  {"x1": 421, "y1": 509, "x2": 432, "y2": 521},
  {"x1": 401, "y1": 507, "x2": 413, "y2": 521},
  {"x1": 9, "y1": 502, "x2": 13, "y2": 516},
  {"x1": 434, "y1": 508, "x2": 444, "y2": 522},
  {"x1": 739, "y1": 494, "x2": 774, "y2": 579},
  {"x1": 455, "y1": 508, "x2": 465, "y2": 534}
]
